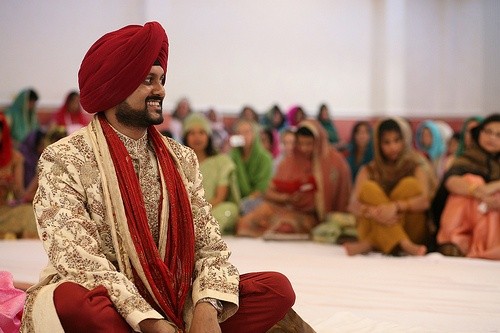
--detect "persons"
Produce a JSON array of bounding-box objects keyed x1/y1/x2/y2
[
  {"x1": 154, "y1": 97, "x2": 500, "y2": 263},
  {"x1": 0, "y1": 89, "x2": 98, "y2": 240},
  {"x1": 17, "y1": 21, "x2": 296, "y2": 333}
]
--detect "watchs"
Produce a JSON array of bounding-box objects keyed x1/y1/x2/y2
[{"x1": 197, "y1": 298, "x2": 224, "y2": 316}]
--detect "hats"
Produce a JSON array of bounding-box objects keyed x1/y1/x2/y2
[{"x1": 78, "y1": 21, "x2": 169, "y2": 113}]
